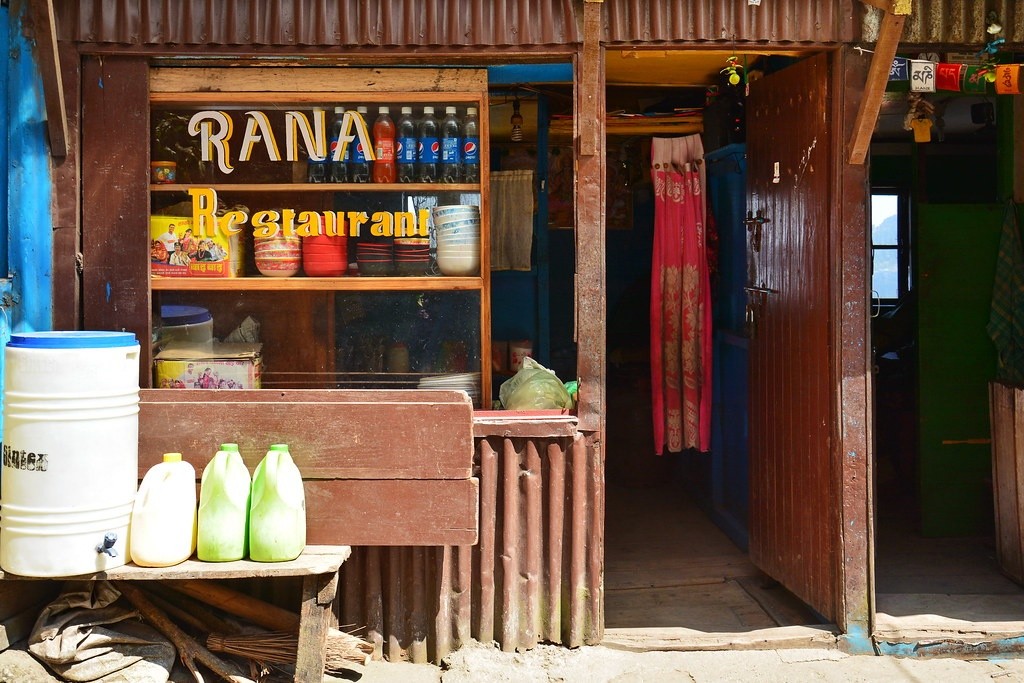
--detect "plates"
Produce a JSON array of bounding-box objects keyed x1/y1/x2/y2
[{"x1": 417, "y1": 372, "x2": 482, "y2": 409}]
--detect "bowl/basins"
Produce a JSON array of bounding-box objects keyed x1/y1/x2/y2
[{"x1": 254, "y1": 205, "x2": 481, "y2": 278}]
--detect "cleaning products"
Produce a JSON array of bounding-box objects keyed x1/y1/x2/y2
[
  {"x1": 128, "y1": 453, "x2": 197, "y2": 567},
  {"x1": 249, "y1": 443, "x2": 306, "y2": 563},
  {"x1": 197, "y1": 443, "x2": 252, "y2": 563}
]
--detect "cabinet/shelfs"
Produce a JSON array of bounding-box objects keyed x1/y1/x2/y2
[{"x1": 147, "y1": 92, "x2": 495, "y2": 411}]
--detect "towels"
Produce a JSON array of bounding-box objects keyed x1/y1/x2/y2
[{"x1": 489, "y1": 169, "x2": 534, "y2": 272}]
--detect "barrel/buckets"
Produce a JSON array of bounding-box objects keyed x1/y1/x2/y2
[
  {"x1": 160, "y1": 304, "x2": 214, "y2": 370},
  {"x1": 1, "y1": 330, "x2": 142, "y2": 578}
]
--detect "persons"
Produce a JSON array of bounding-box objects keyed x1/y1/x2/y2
[
  {"x1": 150, "y1": 223, "x2": 227, "y2": 267},
  {"x1": 160, "y1": 363, "x2": 244, "y2": 390}
]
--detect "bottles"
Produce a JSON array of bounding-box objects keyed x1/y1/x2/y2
[
  {"x1": 150, "y1": 161, "x2": 176, "y2": 184},
  {"x1": 130, "y1": 443, "x2": 307, "y2": 568},
  {"x1": 307, "y1": 105, "x2": 480, "y2": 183}
]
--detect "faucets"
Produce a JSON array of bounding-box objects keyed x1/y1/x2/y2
[{"x1": 95, "y1": 532, "x2": 118, "y2": 558}]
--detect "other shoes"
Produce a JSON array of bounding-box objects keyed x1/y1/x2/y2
[{"x1": 877, "y1": 459, "x2": 897, "y2": 481}]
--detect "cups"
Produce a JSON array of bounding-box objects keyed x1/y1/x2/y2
[{"x1": 335, "y1": 336, "x2": 534, "y2": 379}]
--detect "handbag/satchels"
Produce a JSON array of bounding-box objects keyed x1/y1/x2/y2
[{"x1": 499, "y1": 357, "x2": 576, "y2": 410}]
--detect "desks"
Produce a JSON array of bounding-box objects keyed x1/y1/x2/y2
[{"x1": 0, "y1": 542, "x2": 352, "y2": 683}]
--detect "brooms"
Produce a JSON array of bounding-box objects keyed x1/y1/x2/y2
[{"x1": 190, "y1": 623, "x2": 375, "y2": 677}]
